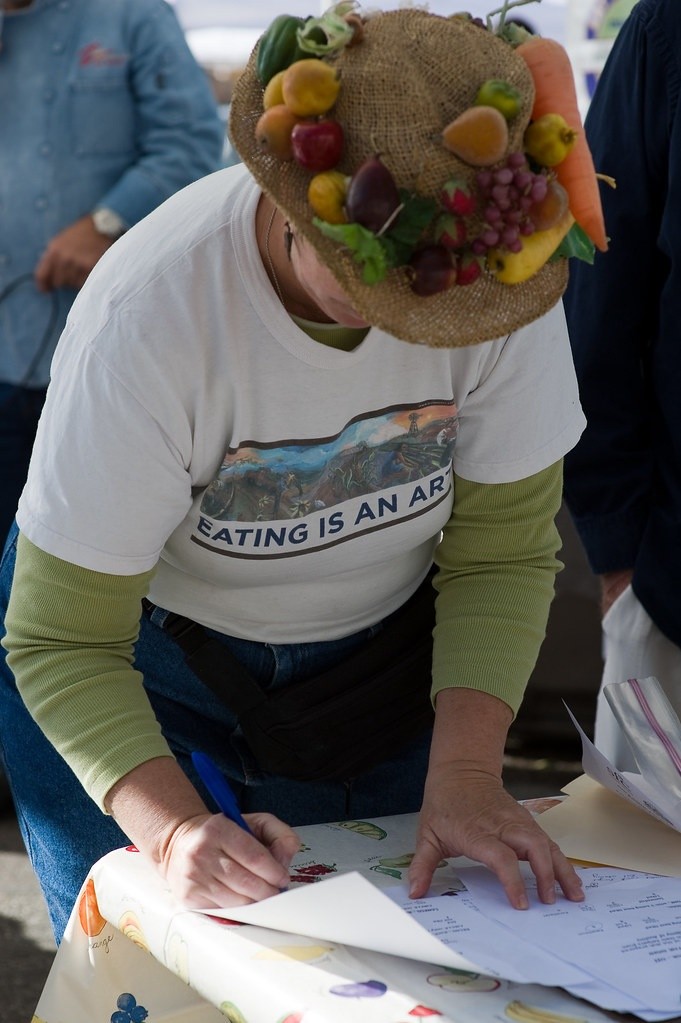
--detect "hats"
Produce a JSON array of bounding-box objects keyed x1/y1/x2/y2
[{"x1": 228, "y1": 0, "x2": 607, "y2": 350}]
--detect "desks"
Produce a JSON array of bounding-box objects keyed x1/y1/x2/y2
[{"x1": 28, "y1": 792, "x2": 681, "y2": 1023}]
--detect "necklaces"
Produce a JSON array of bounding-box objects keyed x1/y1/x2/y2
[{"x1": 262, "y1": 206, "x2": 285, "y2": 305}]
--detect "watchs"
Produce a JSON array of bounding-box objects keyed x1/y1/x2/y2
[{"x1": 92, "y1": 206, "x2": 125, "y2": 241}]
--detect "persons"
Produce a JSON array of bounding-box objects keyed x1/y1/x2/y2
[
  {"x1": 0, "y1": 0, "x2": 229, "y2": 546},
  {"x1": 562, "y1": 0, "x2": 681, "y2": 775},
  {"x1": 0, "y1": 0, "x2": 610, "y2": 947}
]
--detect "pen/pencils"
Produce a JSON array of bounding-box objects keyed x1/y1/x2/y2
[{"x1": 192, "y1": 749, "x2": 253, "y2": 833}]
